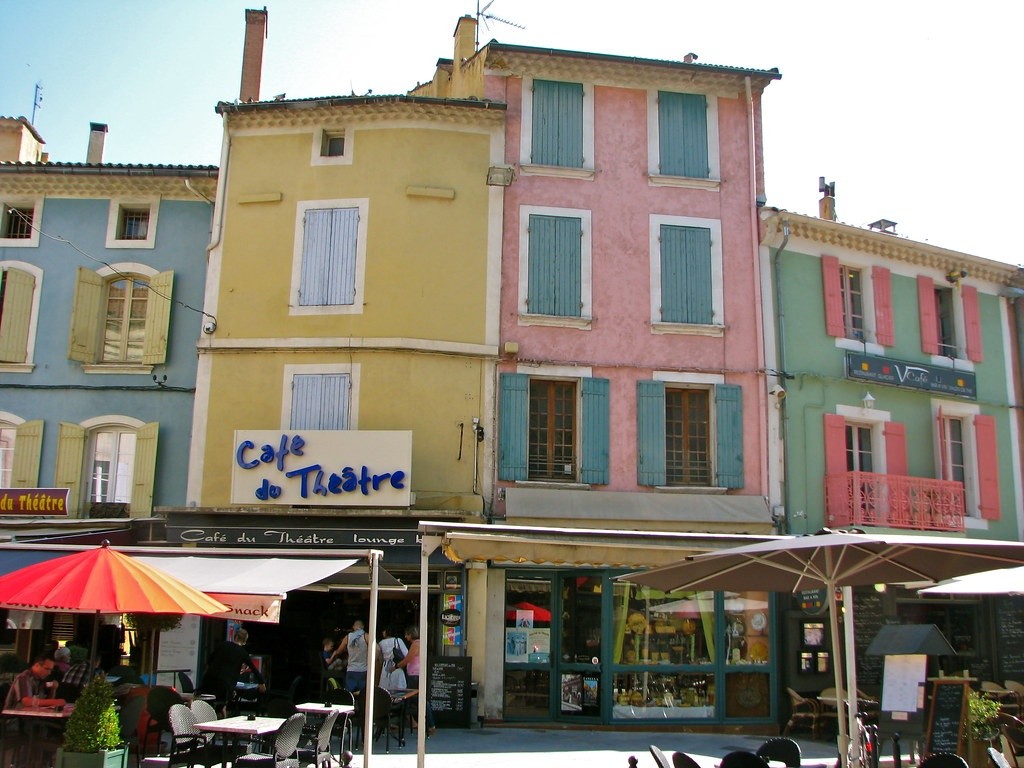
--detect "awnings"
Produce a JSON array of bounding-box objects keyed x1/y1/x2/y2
[
  {"x1": 0, "y1": 548, "x2": 359, "y2": 623},
  {"x1": 505, "y1": 487, "x2": 775, "y2": 535},
  {"x1": 442, "y1": 530, "x2": 755, "y2": 570}
]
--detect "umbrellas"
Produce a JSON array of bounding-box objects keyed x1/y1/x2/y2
[
  {"x1": 505, "y1": 602, "x2": 551, "y2": 622},
  {"x1": 641, "y1": 590, "x2": 768, "y2": 613},
  {"x1": 0, "y1": 540, "x2": 232, "y2": 683},
  {"x1": 918, "y1": 565, "x2": 1024, "y2": 596},
  {"x1": 614, "y1": 527, "x2": 1024, "y2": 768}
]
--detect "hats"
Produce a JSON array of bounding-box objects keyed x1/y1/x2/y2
[{"x1": 54, "y1": 647, "x2": 70, "y2": 659}]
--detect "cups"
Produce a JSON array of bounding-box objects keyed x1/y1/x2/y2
[
  {"x1": 32, "y1": 695, "x2": 40, "y2": 711},
  {"x1": 45, "y1": 682, "x2": 54, "y2": 687}
]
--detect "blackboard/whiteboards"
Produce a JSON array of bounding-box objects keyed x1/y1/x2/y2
[
  {"x1": 427, "y1": 657, "x2": 472, "y2": 725},
  {"x1": 926, "y1": 678, "x2": 967, "y2": 757}
]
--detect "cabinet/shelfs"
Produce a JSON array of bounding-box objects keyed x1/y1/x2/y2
[{"x1": 613, "y1": 608, "x2": 713, "y2": 707}]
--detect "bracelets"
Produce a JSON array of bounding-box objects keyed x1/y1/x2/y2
[{"x1": 395, "y1": 664, "x2": 399, "y2": 669}]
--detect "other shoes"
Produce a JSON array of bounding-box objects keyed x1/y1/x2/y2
[
  {"x1": 427, "y1": 727, "x2": 437, "y2": 735},
  {"x1": 412, "y1": 725, "x2": 418, "y2": 729}
]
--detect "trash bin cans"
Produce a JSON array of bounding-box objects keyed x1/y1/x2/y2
[{"x1": 472, "y1": 683, "x2": 479, "y2": 722}]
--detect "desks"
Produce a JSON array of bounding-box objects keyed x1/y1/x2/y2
[
  {"x1": 294, "y1": 702, "x2": 354, "y2": 752},
  {"x1": 194, "y1": 715, "x2": 288, "y2": 768},
  {"x1": 179, "y1": 693, "x2": 215, "y2": 707},
  {"x1": 2, "y1": 706, "x2": 72, "y2": 768},
  {"x1": 816, "y1": 697, "x2": 848, "y2": 735},
  {"x1": 354, "y1": 688, "x2": 420, "y2": 744}
]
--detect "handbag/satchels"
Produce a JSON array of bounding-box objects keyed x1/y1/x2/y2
[{"x1": 393, "y1": 637, "x2": 404, "y2": 664}]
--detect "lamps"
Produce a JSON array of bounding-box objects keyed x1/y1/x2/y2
[
  {"x1": 999, "y1": 286, "x2": 1024, "y2": 300},
  {"x1": 946, "y1": 268, "x2": 967, "y2": 286},
  {"x1": 485, "y1": 163, "x2": 514, "y2": 187},
  {"x1": 862, "y1": 391, "x2": 875, "y2": 415}
]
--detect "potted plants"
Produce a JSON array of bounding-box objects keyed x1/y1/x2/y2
[
  {"x1": 966, "y1": 691, "x2": 1007, "y2": 754},
  {"x1": 55, "y1": 671, "x2": 128, "y2": 768}
]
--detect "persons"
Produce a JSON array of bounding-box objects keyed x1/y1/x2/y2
[
  {"x1": 2, "y1": 654, "x2": 66, "y2": 768},
  {"x1": 44, "y1": 640, "x2": 108, "y2": 689},
  {"x1": 322, "y1": 620, "x2": 437, "y2": 735},
  {"x1": 200, "y1": 628, "x2": 266, "y2": 740}
]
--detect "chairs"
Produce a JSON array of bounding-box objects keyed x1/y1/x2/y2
[
  {"x1": 0, "y1": 652, "x2": 410, "y2": 768},
  {"x1": 650, "y1": 680, "x2": 1024, "y2": 768}
]
